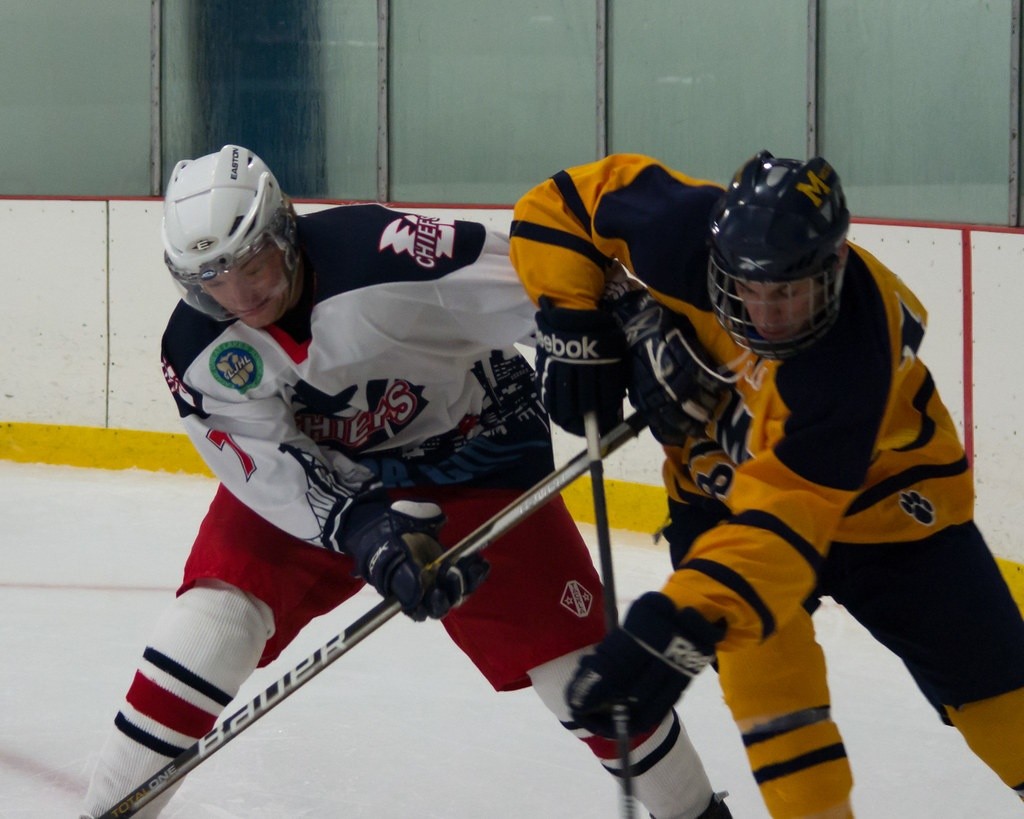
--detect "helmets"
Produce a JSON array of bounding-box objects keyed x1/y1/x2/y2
[
  {"x1": 160, "y1": 143, "x2": 283, "y2": 275},
  {"x1": 711, "y1": 146, "x2": 854, "y2": 281}
]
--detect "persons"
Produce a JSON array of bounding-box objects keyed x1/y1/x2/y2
[
  {"x1": 506, "y1": 150, "x2": 1024, "y2": 818},
  {"x1": 77, "y1": 145, "x2": 733, "y2": 818}
]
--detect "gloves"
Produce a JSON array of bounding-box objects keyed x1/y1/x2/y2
[
  {"x1": 614, "y1": 294, "x2": 734, "y2": 452},
  {"x1": 561, "y1": 591, "x2": 736, "y2": 747},
  {"x1": 335, "y1": 501, "x2": 490, "y2": 624},
  {"x1": 530, "y1": 297, "x2": 626, "y2": 441}
]
